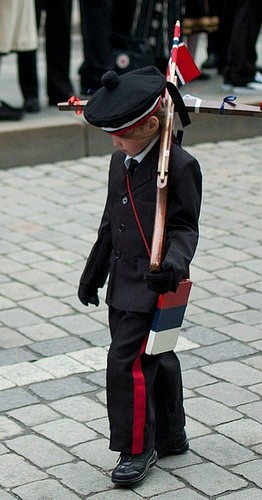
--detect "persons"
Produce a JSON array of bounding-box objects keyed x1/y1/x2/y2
[
  {"x1": 0, "y1": 0, "x2": 262, "y2": 119},
  {"x1": 78, "y1": 66, "x2": 203, "y2": 485}
]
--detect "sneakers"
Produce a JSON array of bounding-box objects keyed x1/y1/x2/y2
[
  {"x1": 112, "y1": 448, "x2": 157, "y2": 483},
  {"x1": 155, "y1": 435, "x2": 188, "y2": 455}
]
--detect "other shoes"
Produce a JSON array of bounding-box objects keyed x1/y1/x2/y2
[
  {"x1": 24, "y1": 98, "x2": 40, "y2": 111},
  {"x1": 224, "y1": 83, "x2": 261, "y2": 95},
  {"x1": 49, "y1": 94, "x2": 73, "y2": 104},
  {"x1": 0, "y1": 103, "x2": 22, "y2": 120}
]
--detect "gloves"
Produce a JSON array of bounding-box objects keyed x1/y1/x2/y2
[
  {"x1": 144, "y1": 267, "x2": 175, "y2": 294},
  {"x1": 78, "y1": 283, "x2": 99, "y2": 307}
]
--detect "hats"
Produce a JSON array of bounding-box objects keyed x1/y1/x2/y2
[{"x1": 80, "y1": 65, "x2": 192, "y2": 136}]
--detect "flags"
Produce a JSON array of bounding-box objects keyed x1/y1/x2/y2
[{"x1": 167, "y1": 20, "x2": 201, "y2": 88}]
